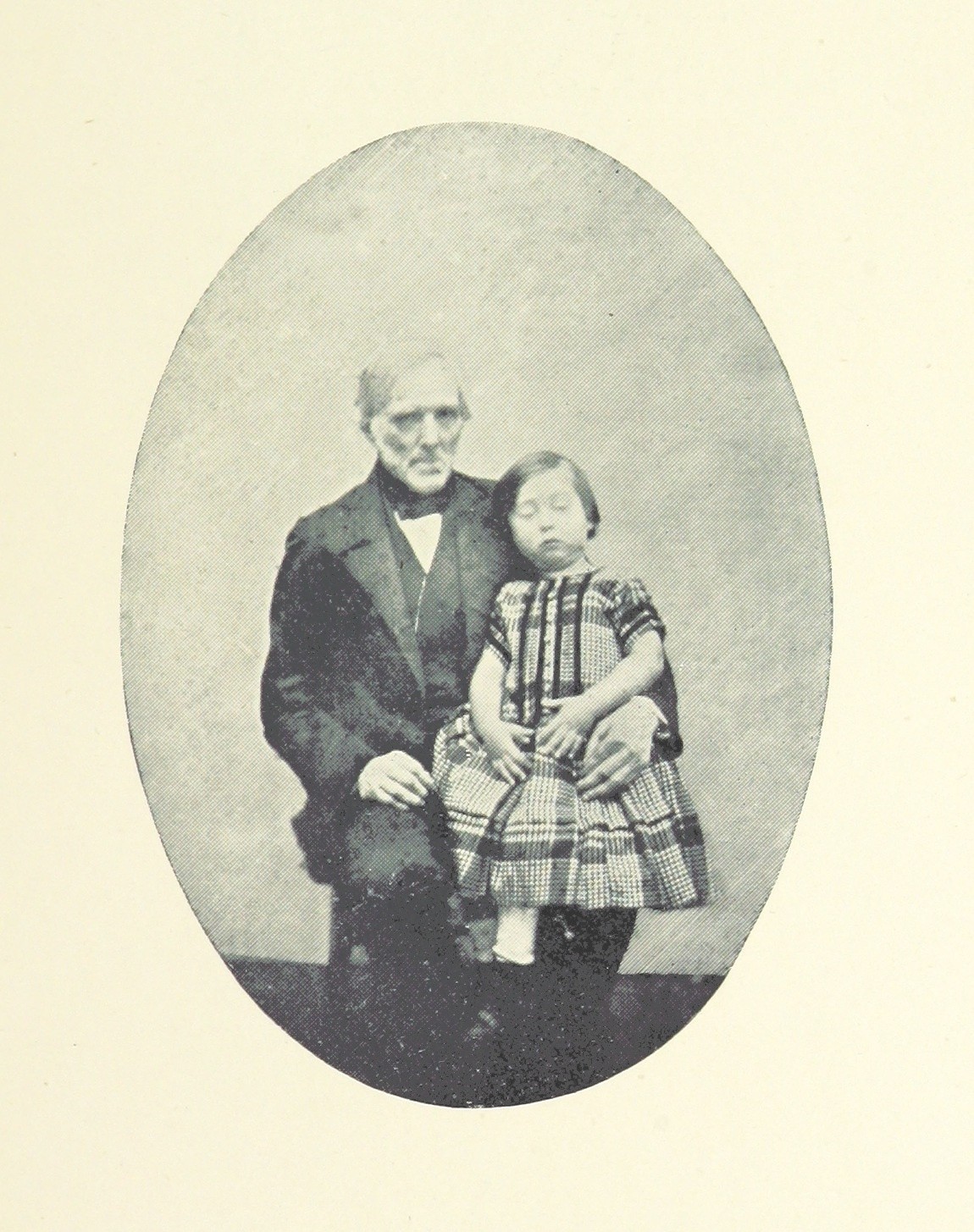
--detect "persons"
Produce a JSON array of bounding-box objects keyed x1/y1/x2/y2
[
  {"x1": 257, "y1": 341, "x2": 678, "y2": 1091},
  {"x1": 433, "y1": 446, "x2": 719, "y2": 1016}
]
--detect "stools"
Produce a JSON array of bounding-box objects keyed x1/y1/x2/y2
[{"x1": 320, "y1": 876, "x2": 383, "y2": 1019}]
